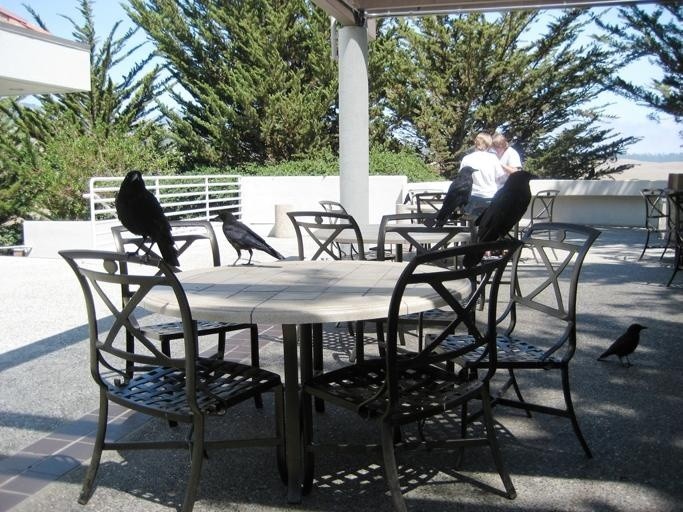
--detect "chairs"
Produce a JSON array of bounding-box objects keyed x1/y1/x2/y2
[
  {"x1": 421, "y1": 221, "x2": 602, "y2": 462},
  {"x1": 58, "y1": 248, "x2": 288, "y2": 509},
  {"x1": 641, "y1": 186, "x2": 683, "y2": 287},
  {"x1": 110, "y1": 219, "x2": 264, "y2": 409},
  {"x1": 297, "y1": 237, "x2": 524, "y2": 512},
  {"x1": 286, "y1": 189, "x2": 562, "y2": 338}
]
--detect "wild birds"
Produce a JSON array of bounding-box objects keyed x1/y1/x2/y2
[
  {"x1": 216, "y1": 211, "x2": 286, "y2": 266},
  {"x1": 462, "y1": 171, "x2": 540, "y2": 268},
  {"x1": 596, "y1": 323, "x2": 648, "y2": 369},
  {"x1": 435, "y1": 166, "x2": 479, "y2": 230},
  {"x1": 115, "y1": 170, "x2": 180, "y2": 267}
]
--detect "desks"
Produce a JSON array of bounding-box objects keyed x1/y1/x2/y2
[{"x1": 126, "y1": 260, "x2": 473, "y2": 509}]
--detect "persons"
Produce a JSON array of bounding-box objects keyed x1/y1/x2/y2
[
  {"x1": 457, "y1": 131, "x2": 506, "y2": 257},
  {"x1": 489, "y1": 134, "x2": 524, "y2": 191}
]
